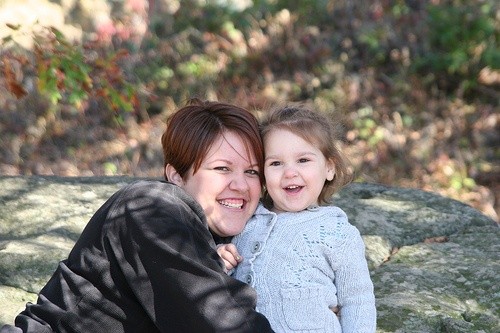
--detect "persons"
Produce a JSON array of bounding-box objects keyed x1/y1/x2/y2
[
  {"x1": 216, "y1": 107, "x2": 377, "y2": 333},
  {"x1": 0, "y1": 100, "x2": 274, "y2": 333}
]
